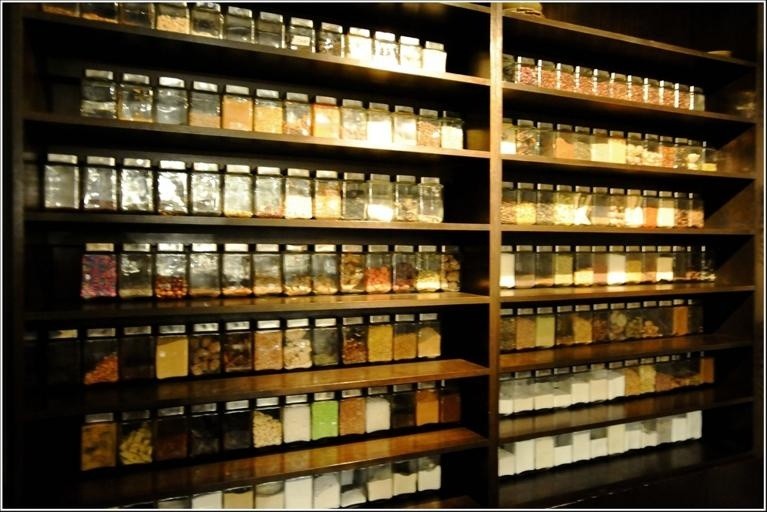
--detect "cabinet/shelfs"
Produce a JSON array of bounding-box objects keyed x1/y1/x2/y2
[
  {"x1": 496, "y1": 5, "x2": 765, "y2": 510},
  {"x1": 1, "y1": 1, "x2": 497, "y2": 510}
]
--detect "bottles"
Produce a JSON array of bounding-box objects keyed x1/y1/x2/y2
[
  {"x1": 503, "y1": 54, "x2": 706, "y2": 110},
  {"x1": 42, "y1": 3, "x2": 448, "y2": 72},
  {"x1": 34, "y1": 150, "x2": 446, "y2": 222},
  {"x1": 499, "y1": 245, "x2": 717, "y2": 288},
  {"x1": 81, "y1": 379, "x2": 462, "y2": 477},
  {"x1": 500, "y1": 299, "x2": 704, "y2": 351},
  {"x1": 502, "y1": 116, "x2": 718, "y2": 172},
  {"x1": 498, "y1": 347, "x2": 715, "y2": 416},
  {"x1": 108, "y1": 452, "x2": 443, "y2": 509},
  {"x1": 498, "y1": 410, "x2": 703, "y2": 479},
  {"x1": 81, "y1": 242, "x2": 461, "y2": 300},
  {"x1": 80, "y1": 68, "x2": 463, "y2": 148},
  {"x1": 501, "y1": 181, "x2": 705, "y2": 229},
  {"x1": 47, "y1": 313, "x2": 443, "y2": 388}
]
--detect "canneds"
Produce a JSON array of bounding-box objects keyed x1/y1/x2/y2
[
  {"x1": 40, "y1": 2, "x2": 448, "y2": 73},
  {"x1": 502, "y1": 51, "x2": 705, "y2": 113},
  {"x1": 501, "y1": 118, "x2": 718, "y2": 173},
  {"x1": 47, "y1": 311, "x2": 443, "y2": 390},
  {"x1": 80, "y1": 241, "x2": 464, "y2": 302},
  {"x1": 79, "y1": 379, "x2": 463, "y2": 474},
  {"x1": 80, "y1": 69, "x2": 466, "y2": 150},
  {"x1": 499, "y1": 296, "x2": 703, "y2": 354},
  {"x1": 157, "y1": 451, "x2": 444, "y2": 509},
  {"x1": 42, "y1": 152, "x2": 444, "y2": 224},
  {"x1": 499, "y1": 352, "x2": 716, "y2": 417},
  {"x1": 499, "y1": 410, "x2": 703, "y2": 478},
  {"x1": 501, "y1": 181, "x2": 704, "y2": 226},
  {"x1": 500, "y1": 242, "x2": 718, "y2": 289}
]
